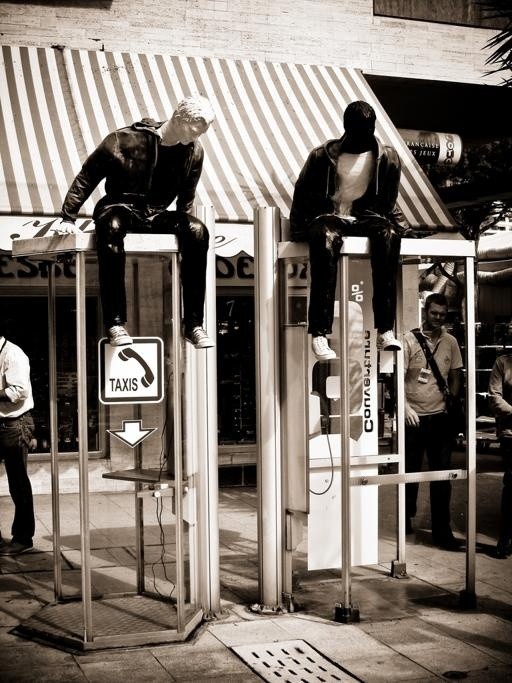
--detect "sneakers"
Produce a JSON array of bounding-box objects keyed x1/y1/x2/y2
[
  {"x1": 379, "y1": 330, "x2": 401, "y2": 351},
  {"x1": 312, "y1": 336, "x2": 337, "y2": 361},
  {"x1": 107, "y1": 325, "x2": 133, "y2": 346},
  {"x1": 183, "y1": 325, "x2": 214, "y2": 348},
  {"x1": 0, "y1": 541, "x2": 33, "y2": 556}
]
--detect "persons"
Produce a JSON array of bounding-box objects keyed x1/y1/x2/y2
[
  {"x1": 488, "y1": 353, "x2": 512, "y2": 556},
  {"x1": 404, "y1": 293, "x2": 466, "y2": 546},
  {"x1": 289, "y1": 101, "x2": 401, "y2": 362},
  {"x1": 0, "y1": 335, "x2": 36, "y2": 557},
  {"x1": 57, "y1": 94, "x2": 216, "y2": 348}
]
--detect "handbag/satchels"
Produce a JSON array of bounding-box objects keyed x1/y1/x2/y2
[{"x1": 445, "y1": 390, "x2": 465, "y2": 433}]
